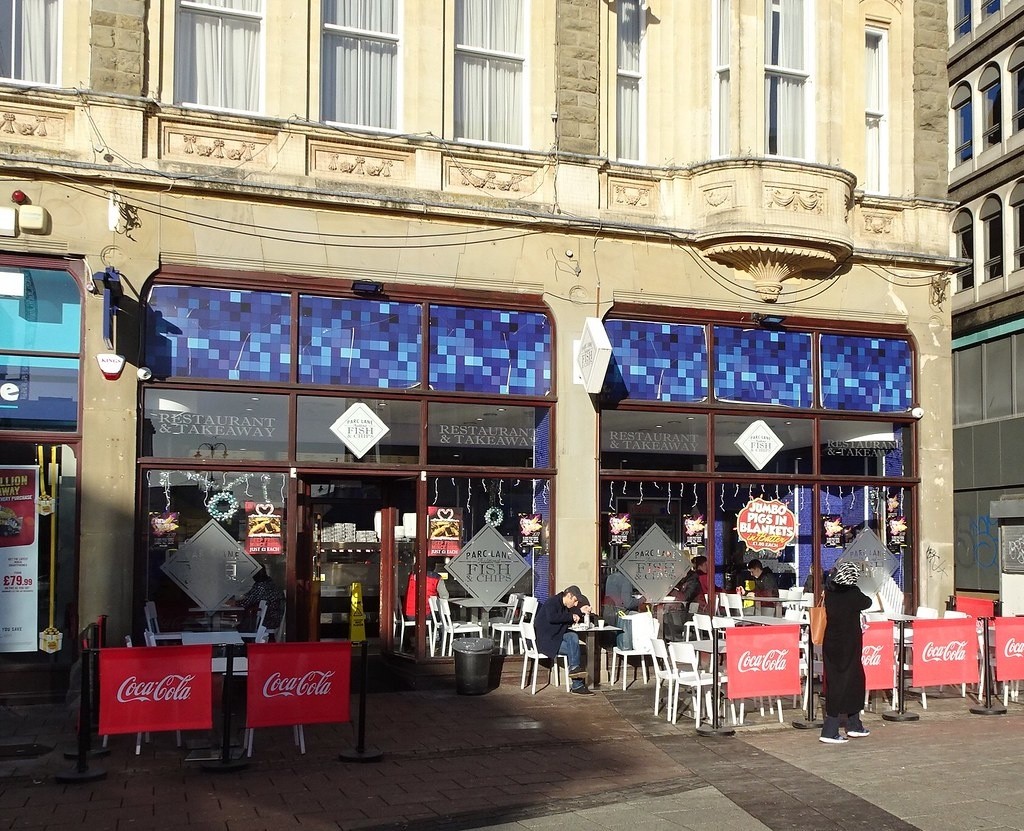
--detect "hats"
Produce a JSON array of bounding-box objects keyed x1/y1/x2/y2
[{"x1": 569, "y1": 586, "x2": 583, "y2": 602}]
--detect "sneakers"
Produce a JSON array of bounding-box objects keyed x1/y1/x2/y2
[
  {"x1": 819, "y1": 732, "x2": 849, "y2": 743},
  {"x1": 571, "y1": 686, "x2": 595, "y2": 696},
  {"x1": 568, "y1": 667, "x2": 588, "y2": 678},
  {"x1": 847, "y1": 726, "x2": 871, "y2": 737}
]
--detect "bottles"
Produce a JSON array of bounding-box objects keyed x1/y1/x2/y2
[{"x1": 584, "y1": 613, "x2": 590, "y2": 628}]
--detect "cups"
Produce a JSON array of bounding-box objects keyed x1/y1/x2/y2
[{"x1": 598, "y1": 620, "x2": 604, "y2": 629}]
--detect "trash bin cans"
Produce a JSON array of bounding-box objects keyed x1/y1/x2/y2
[{"x1": 451, "y1": 637, "x2": 495, "y2": 695}]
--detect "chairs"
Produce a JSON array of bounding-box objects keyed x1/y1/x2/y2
[
  {"x1": 393, "y1": 592, "x2": 539, "y2": 657},
  {"x1": 649, "y1": 586, "x2": 1020, "y2": 729},
  {"x1": 519, "y1": 618, "x2": 660, "y2": 695},
  {"x1": 265, "y1": 590, "x2": 286, "y2": 643},
  {"x1": 102, "y1": 600, "x2": 306, "y2": 758}
]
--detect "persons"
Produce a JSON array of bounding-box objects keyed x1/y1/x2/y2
[
  {"x1": 402, "y1": 558, "x2": 450, "y2": 654},
  {"x1": 604, "y1": 556, "x2": 779, "y2": 608},
  {"x1": 166, "y1": 563, "x2": 281, "y2": 629},
  {"x1": 818, "y1": 560, "x2": 873, "y2": 744},
  {"x1": 533, "y1": 586, "x2": 595, "y2": 696}
]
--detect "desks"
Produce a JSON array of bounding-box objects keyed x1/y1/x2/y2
[
  {"x1": 741, "y1": 596, "x2": 808, "y2": 618},
  {"x1": 189, "y1": 604, "x2": 245, "y2": 631},
  {"x1": 181, "y1": 631, "x2": 256, "y2": 772},
  {"x1": 732, "y1": 616, "x2": 810, "y2": 626},
  {"x1": 666, "y1": 640, "x2": 726, "y2": 653},
  {"x1": 448, "y1": 598, "x2": 514, "y2": 639},
  {"x1": 644, "y1": 600, "x2": 682, "y2": 666},
  {"x1": 567, "y1": 625, "x2": 622, "y2": 690}
]
